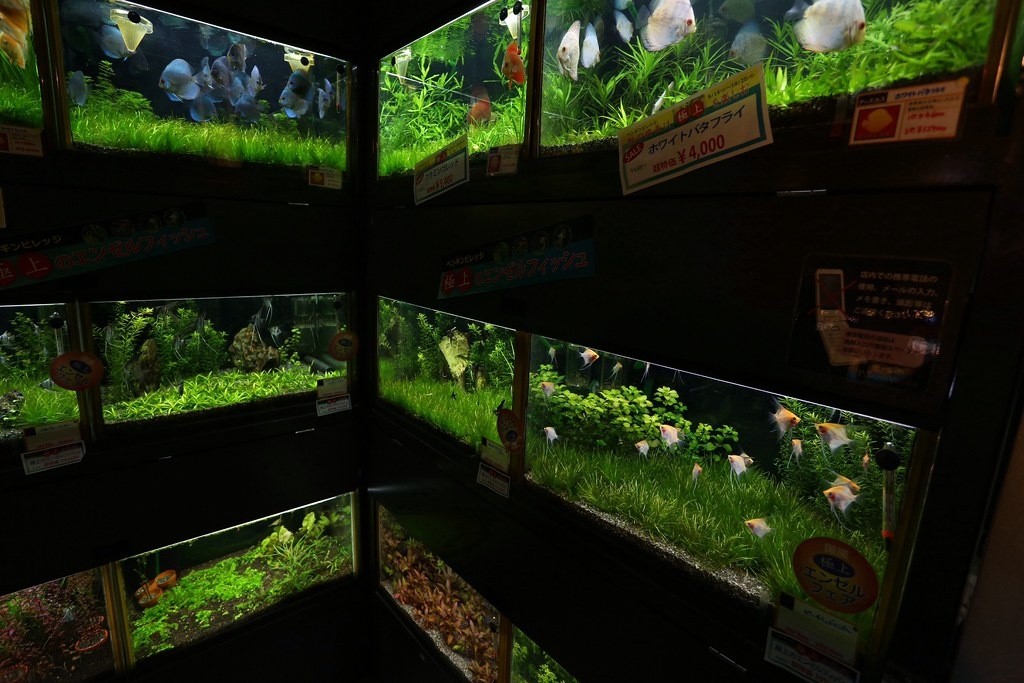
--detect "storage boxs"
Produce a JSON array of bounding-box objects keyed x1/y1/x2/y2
[{"x1": 0, "y1": 0, "x2": 1024, "y2": 683}]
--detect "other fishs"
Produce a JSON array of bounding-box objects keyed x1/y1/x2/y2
[
  {"x1": 660, "y1": 425, "x2": 686, "y2": 451},
  {"x1": 822, "y1": 470, "x2": 863, "y2": 532},
  {"x1": 541, "y1": 382, "x2": 554, "y2": 397},
  {"x1": 67, "y1": 70, "x2": 87, "y2": 107},
  {"x1": 815, "y1": 422, "x2": 855, "y2": 460},
  {"x1": 795, "y1": 0, "x2": 866, "y2": 53},
  {"x1": 614, "y1": 0, "x2": 635, "y2": 42},
  {"x1": 579, "y1": 347, "x2": 600, "y2": 370},
  {"x1": 729, "y1": 18, "x2": 767, "y2": 65},
  {"x1": 466, "y1": 96, "x2": 491, "y2": 125},
  {"x1": 609, "y1": 362, "x2": 623, "y2": 381},
  {"x1": 549, "y1": 347, "x2": 556, "y2": 364},
  {"x1": 544, "y1": 427, "x2": 559, "y2": 442},
  {"x1": 863, "y1": 453, "x2": 870, "y2": 468},
  {"x1": 556, "y1": 19, "x2": 581, "y2": 81},
  {"x1": 640, "y1": 0, "x2": 697, "y2": 52},
  {"x1": 157, "y1": 43, "x2": 334, "y2": 122},
  {"x1": 634, "y1": 438, "x2": 651, "y2": 461},
  {"x1": 692, "y1": 462, "x2": 703, "y2": 481},
  {"x1": 0, "y1": 0, "x2": 33, "y2": 69},
  {"x1": 744, "y1": 518, "x2": 775, "y2": 538},
  {"x1": 582, "y1": 22, "x2": 601, "y2": 68},
  {"x1": 769, "y1": 399, "x2": 801, "y2": 440},
  {"x1": 791, "y1": 438, "x2": 804, "y2": 462},
  {"x1": 501, "y1": 41, "x2": 525, "y2": 89},
  {"x1": 728, "y1": 452, "x2": 754, "y2": 490}
]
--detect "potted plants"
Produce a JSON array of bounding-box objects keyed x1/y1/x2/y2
[
  {"x1": 137, "y1": 581, "x2": 164, "y2": 611},
  {"x1": 134, "y1": 555, "x2": 158, "y2": 601},
  {"x1": 0, "y1": 664, "x2": 27, "y2": 683},
  {"x1": 0, "y1": 650, "x2": 22, "y2": 666},
  {"x1": 154, "y1": 569, "x2": 177, "y2": 592},
  {"x1": 75, "y1": 609, "x2": 105, "y2": 636},
  {"x1": 75, "y1": 629, "x2": 108, "y2": 664}
]
